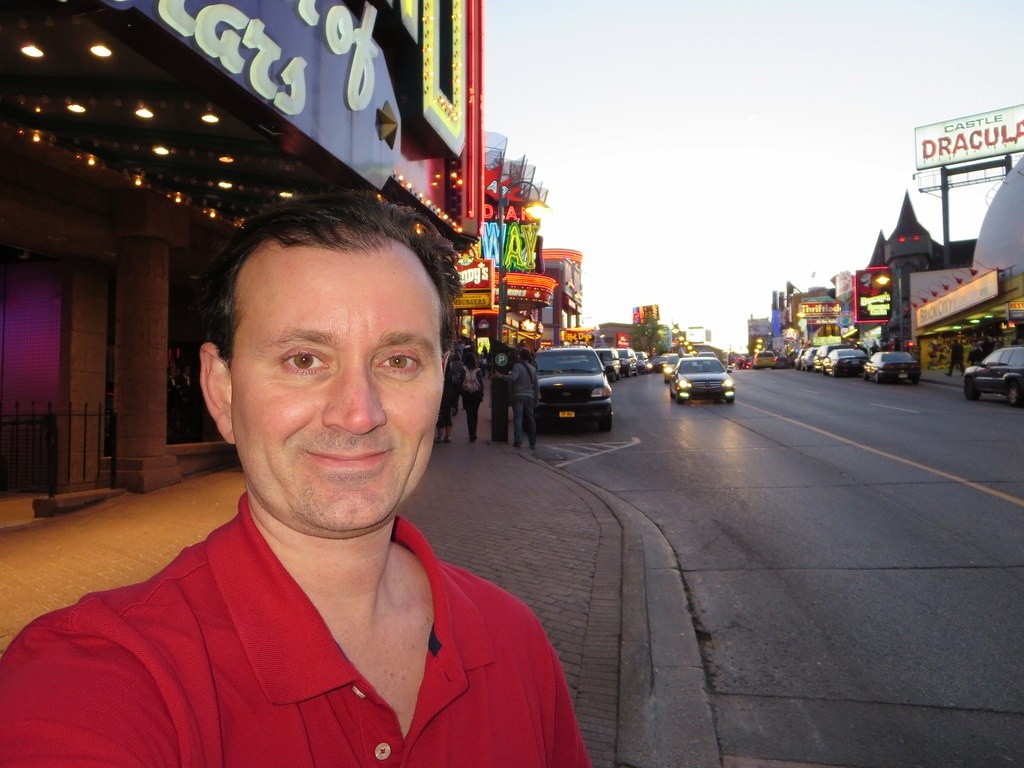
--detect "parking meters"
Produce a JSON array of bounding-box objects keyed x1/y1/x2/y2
[{"x1": 488, "y1": 334, "x2": 516, "y2": 443}]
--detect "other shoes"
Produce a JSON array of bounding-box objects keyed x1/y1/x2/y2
[
  {"x1": 513, "y1": 442, "x2": 521, "y2": 447},
  {"x1": 444, "y1": 436, "x2": 452, "y2": 442},
  {"x1": 435, "y1": 436, "x2": 444, "y2": 442},
  {"x1": 529, "y1": 443, "x2": 535, "y2": 447}
]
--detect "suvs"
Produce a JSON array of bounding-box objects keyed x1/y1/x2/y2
[
  {"x1": 592, "y1": 348, "x2": 621, "y2": 383},
  {"x1": 635, "y1": 351, "x2": 651, "y2": 375},
  {"x1": 616, "y1": 347, "x2": 638, "y2": 378},
  {"x1": 531, "y1": 344, "x2": 613, "y2": 434}
]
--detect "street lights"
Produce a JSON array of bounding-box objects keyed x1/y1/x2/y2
[
  {"x1": 496, "y1": 178, "x2": 553, "y2": 345},
  {"x1": 876, "y1": 267, "x2": 904, "y2": 352},
  {"x1": 796, "y1": 311, "x2": 813, "y2": 348}
]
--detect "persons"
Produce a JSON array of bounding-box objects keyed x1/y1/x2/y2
[
  {"x1": 449, "y1": 351, "x2": 464, "y2": 416},
  {"x1": 493, "y1": 347, "x2": 540, "y2": 449},
  {"x1": 945, "y1": 338, "x2": 965, "y2": 376},
  {"x1": 0, "y1": 191, "x2": 592, "y2": 768},
  {"x1": 457, "y1": 353, "x2": 484, "y2": 443},
  {"x1": 871, "y1": 341, "x2": 879, "y2": 354},
  {"x1": 434, "y1": 364, "x2": 454, "y2": 443},
  {"x1": 966, "y1": 337, "x2": 1004, "y2": 366}
]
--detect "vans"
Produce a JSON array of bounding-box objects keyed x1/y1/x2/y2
[{"x1": 652, "y1": 354, "x2": 679, "y2": 373}]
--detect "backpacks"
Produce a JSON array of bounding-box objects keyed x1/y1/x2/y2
[{"x1": 463, "y1": 366, "x2": 481, "y2": 392}]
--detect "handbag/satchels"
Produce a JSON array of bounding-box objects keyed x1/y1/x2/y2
[{"x1": 452, "y1": 372, "x2": 462, "y2": 383}]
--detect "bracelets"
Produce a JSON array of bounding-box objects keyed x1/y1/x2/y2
[{"x1": 499, "y1": 374, "x2": 502, "y2": 378}]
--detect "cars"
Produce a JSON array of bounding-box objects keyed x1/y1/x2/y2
[
  {"x1": 822, "y1": 349, "x2": 870, "y2": 377},
  {"x1": 664, "y1": 353, "x2": 695, "y2": 384},
  {"x1": 863, "y1": 351, "x2": 921, "y2": 384},
  {"x1": 794, "y1": 349, "x2": 807, "y2": 369},
  {"x1": 696, "y1": 352, "x2": 717, "y2": 357},
  {"x1": 800, "y1": 347, "x2": 820, "y2": 371},
  {"x1": 813, "y1": 344, "x2": 853, "y2": 373},
  {"x1": 669, "y1": 357, "x2": 736, "y2": 405},
  {"x1": 753, "y1": 351, "x2": 778, "y2": 369},
  {"x1": 963, "y1": 345, "x2": 1024, "y2": 407}
]
